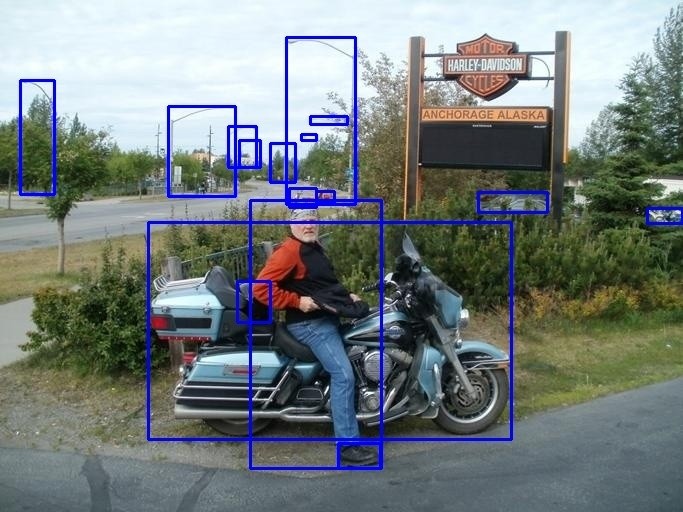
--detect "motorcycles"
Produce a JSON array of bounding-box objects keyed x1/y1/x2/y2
[
  {"x1": 149, "y1": 223, "x2": 512, "y2": 440},
  {"x1": 290, "y1": 188, "x2": 317, "y2": 208},
  {"x1": 317, "y1": 192, "x2": 334, "y2": 203}
]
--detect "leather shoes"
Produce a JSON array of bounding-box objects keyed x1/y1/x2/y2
[{"x1": 339, "y1": 444, "x2": 381, "y2": 468}]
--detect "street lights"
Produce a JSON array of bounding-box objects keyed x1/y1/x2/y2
[
  {"x1": 168, "y1": 106, "x2": 235, "y2": 196},
  {"x1": 240, "y1": 141, "x2": 261, "y2": 168},
  {"x1": 302, "y1": 134, "x2": 317, "y2": 140},
  {"x1": 21, "y1": 80, "x2": 55, "y2": 195},
  {"x1": 228, "y1": 126, "x2": 256, "y2": 167},
  {"x1": 310, "y1": 116, "x2": 347, "y2": 124},
  {"x1": 270, "y1": 144, "x2": 296, "y2": 183},
  {"x1": 286, "y1": 38, "x2": 356, "y2": 205}
]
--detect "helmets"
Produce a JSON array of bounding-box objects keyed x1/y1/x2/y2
[{"x1": 237, "y1": 281, "x2": 270, "y2": 323}]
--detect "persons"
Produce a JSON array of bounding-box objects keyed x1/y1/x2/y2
[{"x1": 251, "y1": 200, "x2": 381, "y2": 469}]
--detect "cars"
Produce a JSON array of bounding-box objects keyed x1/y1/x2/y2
[
  {"x1": 648, "y1": 209, "x2": 683, "y2": 224},
  {"x1": 478, "y1": 192, "x2": 548, "y2": 212}
]
